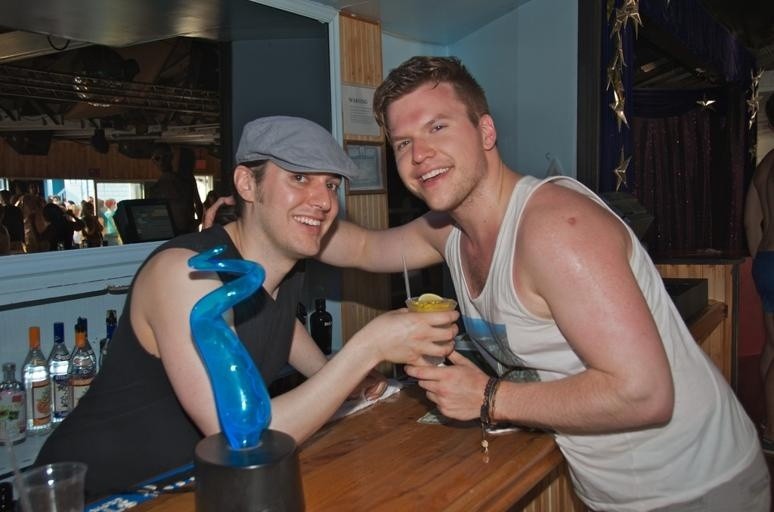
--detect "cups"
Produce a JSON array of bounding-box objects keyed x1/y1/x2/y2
[
  {"x1": 405, "y1": 297, "x2": 458, "y2": 365},
  {"x1": 12, "y1": 461, "x2": 89, "y2": 512}
]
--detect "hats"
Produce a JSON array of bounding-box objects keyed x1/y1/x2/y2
[{"x1": 236, "y1": 116, "x2": 360, "y2": 181}]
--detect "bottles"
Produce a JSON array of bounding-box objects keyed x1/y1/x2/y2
[
  {"x1": 309, "y1": 297, "x2": 333, "y2": 356},
  {"x1": 0, "y1": 309, "x2": 117, "y2": 446}
]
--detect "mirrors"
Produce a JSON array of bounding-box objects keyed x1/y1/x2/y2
[{"x1": 0, "y1": 25, "x2": 227, "y2": 257}]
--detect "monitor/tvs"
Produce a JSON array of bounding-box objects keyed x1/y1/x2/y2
[{"x1": 113, "y1": 196, "x2": 176, "y2": 243}]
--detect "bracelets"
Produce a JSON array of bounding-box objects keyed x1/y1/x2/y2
[{"x1": 480, "y1": 376, "x2": 502, "y2": 453}]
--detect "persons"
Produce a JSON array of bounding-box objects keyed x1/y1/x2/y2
[
  {"x1": 203, "y1": 191, "x2": 218, "y2": 214},
  {"x1": 0, "y1": 180, "x2": 122, "y2": 251},
  {"x1": 204, "y1": 57, "x2": 772, "y2": 512},
  {"x1": 744, "y1": 94, "x2": 774, "y2": 454},
  {"x1": 33, "y1": 116, "x2": 459, "y2": 499}
]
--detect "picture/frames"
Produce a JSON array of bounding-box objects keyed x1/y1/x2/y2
[{"x1": 345, "y1": 140, "x2": 388, "y2": 195}]
